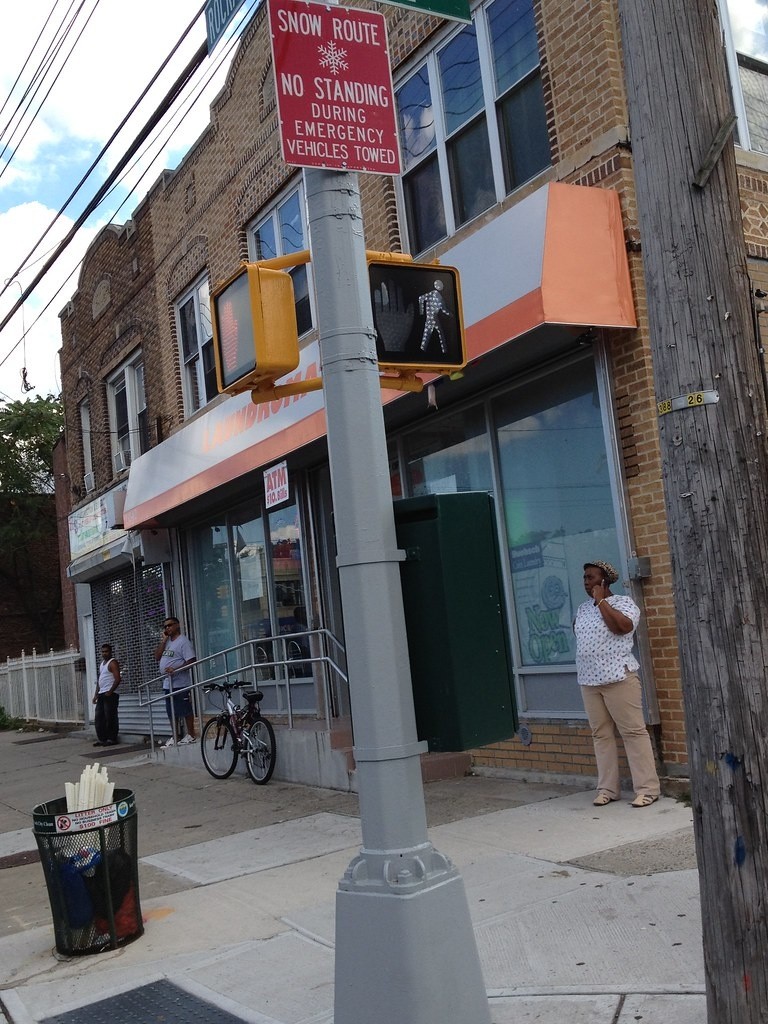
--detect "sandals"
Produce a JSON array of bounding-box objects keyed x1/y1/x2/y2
[
  {"x1": 593, "y1": 794, "x2": 612, "y2": 806},
  {"x1": 632, "y1": 794, "x2": 658, "y2": 806}
]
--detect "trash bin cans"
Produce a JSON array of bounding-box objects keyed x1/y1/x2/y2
[{"x1": 32, "y1": 789, "x2": 145, "y2": 958}]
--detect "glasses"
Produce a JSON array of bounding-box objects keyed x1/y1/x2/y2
[{"x1": 165, "y1": 623, "x2": 177, "y2": 629}]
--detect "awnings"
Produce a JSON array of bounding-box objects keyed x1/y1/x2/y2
[{"x1": 122, "y1": 182, "x2": 638, "y2": 532}]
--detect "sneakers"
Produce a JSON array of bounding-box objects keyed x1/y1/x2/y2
[
  {"x1": 160, "y1": 737, "x2": 181, "y2": 749},
  {"x1": 177, "y1": 734, "x2": 197, "y2": 746}
]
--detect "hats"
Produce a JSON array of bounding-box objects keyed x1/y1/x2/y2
[{"x1": 583, "y1": 560, "x2": 618, "y2": 584}]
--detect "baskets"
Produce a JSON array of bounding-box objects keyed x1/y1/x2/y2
[{"x1": 33, "y1": 788, "x2": 145, "y2": 956}]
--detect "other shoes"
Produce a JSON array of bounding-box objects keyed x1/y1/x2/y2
[{"x1": 93, "y1": 742, "x2": 118, "y2": 746}]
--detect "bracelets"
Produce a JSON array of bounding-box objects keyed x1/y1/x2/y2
[{"x1": 598, "y1": 599, "x2": 605, "y2": 607}]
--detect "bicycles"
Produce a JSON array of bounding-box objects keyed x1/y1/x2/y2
[{"x1": 198, "y1": 679, "x2": 277, "y2": 785}]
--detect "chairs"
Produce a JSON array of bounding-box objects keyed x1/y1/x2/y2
[
  {"x1": 255, "y1": 647, "x2": 271, "y2": 680},
  {"x1": 288, "y1": 641, "x2": 305, "y2": 678}
]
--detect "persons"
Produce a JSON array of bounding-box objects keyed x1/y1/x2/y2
[
  {"x1": 155, "y1": 617, "x2": 197, "y2": 749},
  {"x1": 291, "y1": 607, "x2": 312, "y2": 678},
  {"x1": 92, "y1": 644, "x2": 121, "y2": 747},
  {"x1": 572, "y1": 560, "x2": 661, "y2": 807}
]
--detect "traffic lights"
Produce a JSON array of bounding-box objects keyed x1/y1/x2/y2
[
  {"x1": 368, "y1": 257, "x2": 495, "y2": 381},
  {"x1": 207, "y1": 264, "x2": 297, "y2": 396}
]
